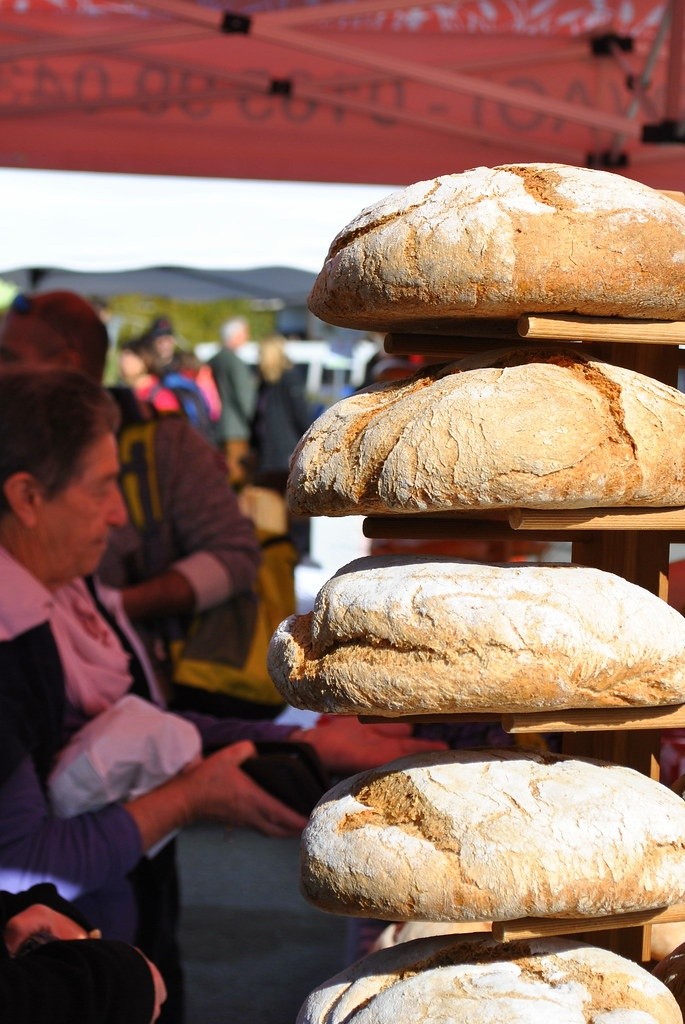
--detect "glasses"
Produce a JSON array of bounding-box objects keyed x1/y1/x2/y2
[{"x1": 8, "y1": 292, "x2": 70, "y2": 337}]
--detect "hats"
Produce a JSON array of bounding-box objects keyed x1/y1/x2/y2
[{"x1": 147, "y1": 315, "x2": 175, "y2": 343}]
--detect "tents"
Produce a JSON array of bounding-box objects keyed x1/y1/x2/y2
[{"x1": 1, "y1": 0, "x2": 685, "y2": 339}]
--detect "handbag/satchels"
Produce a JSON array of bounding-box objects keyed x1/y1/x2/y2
[{"x1": 112, "y1": 422, "x2": 306, "y2": 707}]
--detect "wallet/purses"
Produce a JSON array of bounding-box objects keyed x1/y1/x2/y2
[{"x1": 197, "y1": 737, "x2": 330, "y2": 819}]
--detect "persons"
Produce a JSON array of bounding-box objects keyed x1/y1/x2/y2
[
  {"x1": 0, "y1": 882, "x2": 168, "y2": 1024},
  {"x1": 0, "y1": 368, "x2": 457, "y2": 1024},
  {"x1": 0, "y1": 282, "x2": 684, "y2": 793}
]
